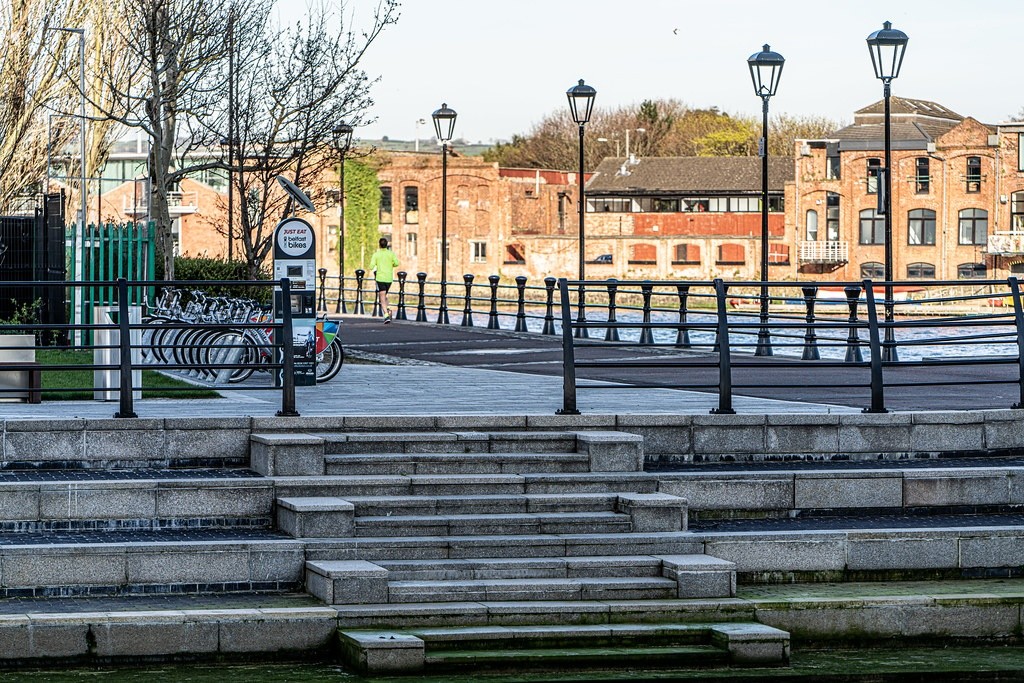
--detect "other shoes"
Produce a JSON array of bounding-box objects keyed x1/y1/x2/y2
[{"x1": 384, "y1": 317, "x2": 391, "y2": 324}]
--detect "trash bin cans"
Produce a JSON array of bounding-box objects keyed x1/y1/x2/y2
[{"x1": 92, "y1": 304, "x2": 145, "y2": 402}]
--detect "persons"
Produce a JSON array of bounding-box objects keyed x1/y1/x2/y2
[{"x1": 369, "y1": 238, "x2": 399, "y2": 323}]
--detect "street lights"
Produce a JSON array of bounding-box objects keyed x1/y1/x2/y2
[
  {"x1": 431, "y1": 102, "x2": 458, "y2": 323},
  {"x1": 866, "y1": 20, "x2": 910, "y2": 367},
  {"x1": 598, "y1": 138, "x2": 620, "y2": 158},
  {"x1": 626, "y1": 127, "x2": 646, "y2": 158},
  {"x1": 746, "y1": 43, "x2": 785, "y2": 357},
  {"x1": 331, "y1": 120, "x2": 354, "y2": 316},
  {"x1": 415, "y1": 118, "x2": 426, "y2": 151},
  {"x1": 564, "y1": 79, "x2": 598, "y2": 340}
]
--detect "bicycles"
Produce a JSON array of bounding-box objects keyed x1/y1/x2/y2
[{"x1": 138, "y1": 284, "x2": 347, "y2": 383}]
